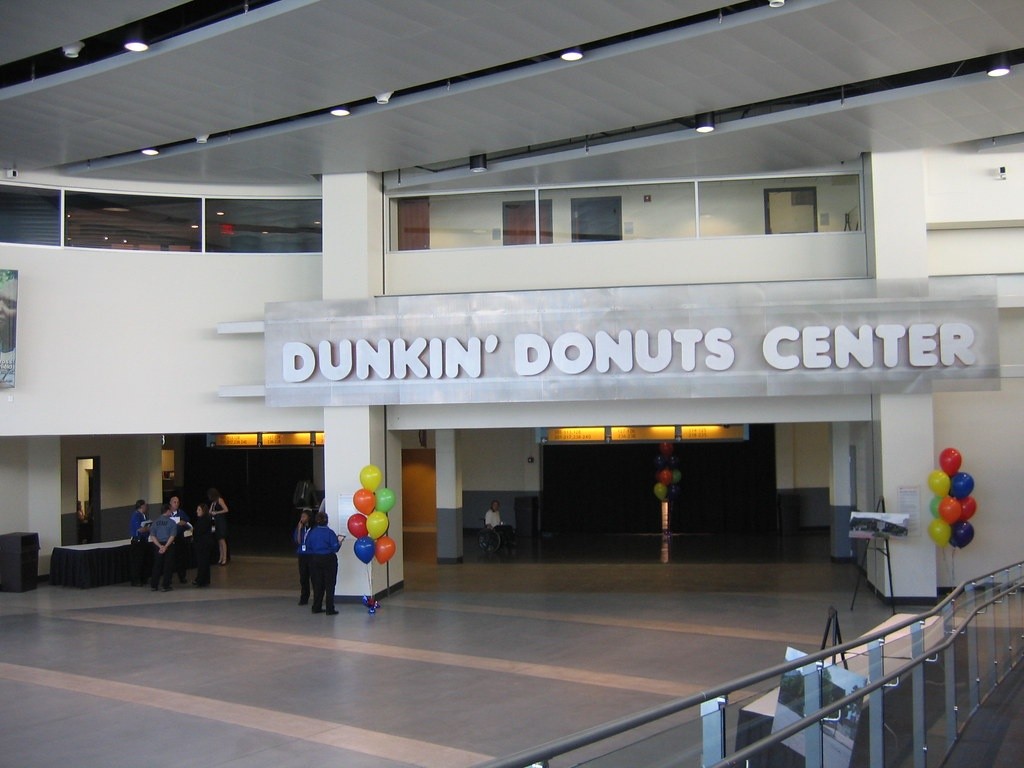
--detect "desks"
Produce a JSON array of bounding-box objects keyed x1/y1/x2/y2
[
  {"x1": 738, "y1": 614, "x2": 970, "y2": 768},
  {"x1": 50, "y1": 527, "x2": 215, "y2": 589}
]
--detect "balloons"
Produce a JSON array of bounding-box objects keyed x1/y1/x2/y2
[
  {"x1": 653, "y1": 442, "x2": 683, "y2": 503},
  {"x1": 929, "y1": 447, "x2": 976, "y2": 550},
  {"x1": 348, "y1": 464, "x2": 396, "y2": 565}
]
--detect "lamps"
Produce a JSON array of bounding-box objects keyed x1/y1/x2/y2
[
  {"x1": 470, "y1": 151, "x2": 487, "y2": 174},
  {"x1": 696, "y1": 110, "x2": 715, "y2": 133},
  {"x1": 144, "y1": 145, "x2": 161, "y2": 156},
  {"x1": 562, "y1": 44, "x2": 584, "y2": 62},
  {"x1": 124, "y1": 19, "x2": 149, "y2": 53},
  {"x1": 331, "y1": 101, "x2": 352, "y2": 117},
  {"x1": 987, "y1": 52, "x2": 1010, "y2": 76}
]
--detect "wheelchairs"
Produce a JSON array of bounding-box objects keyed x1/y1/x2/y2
[{"x1": 478, "y1": 518, "x2": 520, "y2": 554}]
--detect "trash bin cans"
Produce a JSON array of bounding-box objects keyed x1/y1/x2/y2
[
  {"x1": 514, "y1": 496, "x2": 539, "y2": 534},
  {"x1": 0, "y1": 532, "x2": 40, "y2": 592},
  {"x1": 777, "y1": 494, "x2": 799, "y2": 535}
]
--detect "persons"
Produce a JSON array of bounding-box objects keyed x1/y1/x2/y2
[
  {"x1": 294, "y1": 508, "x2": 342, "y2": 616},
  {"x1": 78, "y1": 501, "x2": 85, "y2": 523},
  {"x1": 293, "y1": 475, "x2": 320, "y2": 507},
  {"x1": 150, "y1": 504, "x2": 177, "y2": 592},
  {"x1": 485, "y1": 499, "x2": 517, "y2": 546},
  {"x1": 191, "y1": 503, "x2": 212, "y2": 587},
  {"x1": 129, "y1": 500, "x2": 152, "y2": 586},
  {"x1": 208, "y1": 488, "x2": 229, "y2": 566},
  {"x1": 169, "y1": 496, "x2": 191, "y2": 584}
]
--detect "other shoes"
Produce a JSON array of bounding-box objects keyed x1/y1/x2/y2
[
  {"x1": 161, "y1": 586, "x2": 172, "y2": 592},
  {"x1": 151, "y1": 585, "x2": 158, "y2": 590},
  {"x1": 312, "y1": 609, "x2": 326, "y2": 613},
  {"x1": 326, "y1": 610, "x2": 338, "y2": 615}
]
